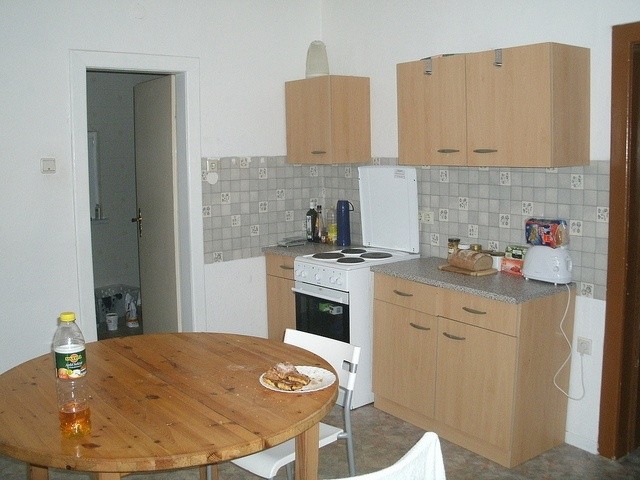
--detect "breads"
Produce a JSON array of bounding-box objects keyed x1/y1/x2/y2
[
  {"x1": 272, "y1": 360, "x2": 311, "y2": 387},
  {"x1": 263, "y1": 369, "x2": 304, "y2": 391}
]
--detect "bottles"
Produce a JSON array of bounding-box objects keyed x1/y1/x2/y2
[
  {"x1": 327, "y1": 205, "x2": 337, "y2": 246},
  {"x1": 336, "y1": 200, "x2": 354, "y2": 246},
  {"x1": 314, "y1": 205, "x2": 324, "y2": 243},
  {"x1": 53, "y1": 312, "x2": 91, "y2": 440},
  {"x1": 306, "y1": 202, "x2": 317, "y2": 242},
  {"x1": 456, "y1": 243, "x2": 471, "y2": 251},
  {"x1": 448, "y1": 238, "x2": 460, "y2": 257},
  {"x1": 489, "y1": 252, "x2": 505, "y2": 271},
  {"x1": 479, "y1": 249, "x2": 495, "y2": 256}
]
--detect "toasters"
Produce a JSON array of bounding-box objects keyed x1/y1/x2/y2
[{"x1": 519, "y1": 244, "x2": 574, "y2": 286}]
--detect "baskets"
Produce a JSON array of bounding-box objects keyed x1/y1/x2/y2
[{"x1": 94, "y1": 283, "x2": 141, "y2": 323}]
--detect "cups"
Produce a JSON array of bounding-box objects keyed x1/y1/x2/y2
[{"x1": 106, "y1": 313, "x2": 119, "y2": 330}]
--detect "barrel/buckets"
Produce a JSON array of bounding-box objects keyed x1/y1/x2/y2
[{"x1": 106, "y1": 312, "x2": 117, "y2": 330}]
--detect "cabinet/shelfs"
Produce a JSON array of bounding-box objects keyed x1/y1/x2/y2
[
  {"x1": 466, "y1": 42, "x2": 590, "y2": 168},
  {"x1": 396, "y1": 55, "x2": 466, "y2": 166},
  {"x1": 285, "y1": 76, "x2": 371, "y2": 165},
  {"x1": 372, "y1": 272, "x2": 576, "y2": 469},
  {"x1": 265, "y1": 253, "x2": 295, "y2": 342}
]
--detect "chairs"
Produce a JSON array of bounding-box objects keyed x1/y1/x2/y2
[
  {"x1": 230, "y1": 326, "x2": 361, "y2": 479},
  {"x1": 342, "y1": 431, "x2": 447, "y2": 480}
]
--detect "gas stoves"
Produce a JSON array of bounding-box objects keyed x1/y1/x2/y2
[{"x1": 295, "y1": 247, "x2": 413, "y2": 270}]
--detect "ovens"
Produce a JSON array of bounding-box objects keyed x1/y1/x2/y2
[{"x1": 291, "y1": 281, "x2": 374, "y2": 411}]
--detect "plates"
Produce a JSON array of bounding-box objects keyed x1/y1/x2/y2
[{"x1": 258, "y1": 365, "x2": 337, "y2": 394}]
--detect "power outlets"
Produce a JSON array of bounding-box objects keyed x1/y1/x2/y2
[{"x1": 575, "y1": 338, "x2": 593, "y2": 356}]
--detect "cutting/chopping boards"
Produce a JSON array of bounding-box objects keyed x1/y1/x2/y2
[{"x1": 438, "y1": 264, "x2": 498, "y2": 277}]
